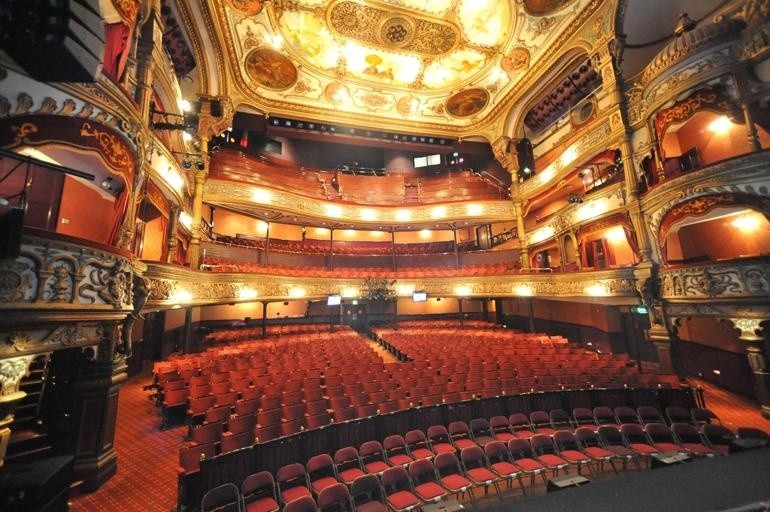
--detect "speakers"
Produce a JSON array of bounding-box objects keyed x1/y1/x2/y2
[
  {"x1": 548, "y1": 474, "x2": 588, "y2": 492},
  {"x1": 729, "y1": 437, "x2": 770, "y2": 452},
  {"x1": 651, "y1": 454, "x2": 691, "y2": 468},
  {"x1": 420, "y1": 498, "x2": 464, "y2": 512}
]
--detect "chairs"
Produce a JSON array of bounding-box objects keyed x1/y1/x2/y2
[
  {"x1": 307, "y1": 454, "x2": 338, "y2": 497},
  {"x1": 200, "y1": 233, "x2": 540, "y2": 279},
  {"x1": 352, "y1": 473, "x2": 390, "y2": 511},
  {"x1": 201, "y1": 483, "x2": 241, "y2": 511},
  {"x1": 434, "y1": 452, "x2": 476, "y2": 512},
  {"x1": 276, "y1": 462, "x2": 313, "y2": 503},
  {"x1": 462, "y1": 446, "x2": 502, "y2": 510},
  {"x1": 241, "y1": 470, "x2": 279, "y2": 511},
  {"x1": 285, "y1": 496, "x2": 317, "y2": 510},
  {"x1": 409, "y1": 457, "x2": 449, "y2": 511},
  {"x1": 181, "y1": 347, "x2": 715, "y2": 463},
  {"x1": 507, "y1": 440, "x2": 549, "y2": 490},
  {"x1": 486, "y1": 441, "x2": 529, "y2": 498},
  {"x1": 365, "y1": 315, "x2": 582, "y2": 362},
  {"x1": 336, "y1": 446, "x2": 365, "y2": 486},
  {"x1": 149, "y1": 321, "x2": 384, "y2": 418},
  {"x1": 317, "y1": 483, "x2": 354, "y2": 511},
  {"x1": 381, "y1": 465, "x2": 423, "y2": 511}
]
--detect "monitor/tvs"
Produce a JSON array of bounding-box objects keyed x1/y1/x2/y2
[
  {"x1": 326, "y1": 295, "x2": 341, "y2": 305},
  {"x1": 413, "y1": 291, "x2": 427, "y2": 302}
]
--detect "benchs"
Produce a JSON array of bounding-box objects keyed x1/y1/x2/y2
[{"x1": 207, "y1": 141, "x2": 509, "y2": 203}]
